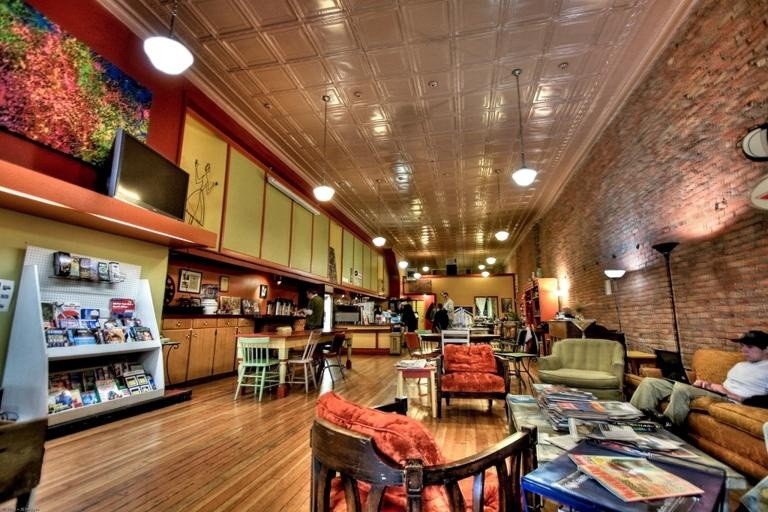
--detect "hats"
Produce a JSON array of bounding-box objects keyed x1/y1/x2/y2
[{"x1": 729, "y1": 329, "x2": 768, "y2": 350}]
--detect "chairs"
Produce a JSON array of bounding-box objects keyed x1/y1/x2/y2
[
  {"x1": 436, "y1": 344, "x2": 511, "y2": 419},
  {"x1": 310, "y1": 395, "x2": 541, "y2": 512},
  {"x1": 441, "y1": 330, "x2": 469, "y2": 356},
  {"x1": 404, "y1": 332, "x2": 440, "y2": 362},
  {"x1": 234, "y1": 330, "x2": 345, "y2": 401},
  {"x1": 537, "y1": 338, "x2": 625, "y2": 401}
]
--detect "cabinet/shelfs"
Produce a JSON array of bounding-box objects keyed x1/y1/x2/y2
[
  {"x1": 523, "y1": 278, "x2": 558, "y2": 354},
  {"x1": 213, "y1": 318, "x2": 255, "y2": 376},
  {"x1": 1, "y1": 246, "x2": 165, "y2": 425},
  {"x1": 160, "y1": 318, "x2": 216, "y2": 386}
]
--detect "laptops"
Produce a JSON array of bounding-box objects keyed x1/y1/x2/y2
[{"x1": 653, "y1": 350, "x2": 727, "y2": 396}]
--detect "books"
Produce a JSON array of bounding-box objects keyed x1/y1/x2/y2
[{"x1": 506, "y1": 381, "x2": 707, "y2": 504}]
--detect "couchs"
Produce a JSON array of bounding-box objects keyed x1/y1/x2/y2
[{"x1": 624, "y1": 349, "x2": 768, "y2": 480}]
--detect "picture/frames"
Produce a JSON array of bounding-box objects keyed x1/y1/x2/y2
[
  {"x1": 219, "y1": 275, "x2": 229, "y2": 292},
  {"x1": 177, "y1": 268, "x2": 202, "y2": 294},
  {"x1": 260, "y1": 285, "x2": 267, "y2": 298}
]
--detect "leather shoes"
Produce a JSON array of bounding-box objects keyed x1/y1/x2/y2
[{"x1": 643, "y1": 408, "x2": 680, "y2": 433}]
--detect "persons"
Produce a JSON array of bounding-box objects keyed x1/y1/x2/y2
[
  {"x1": 401, "y1": 291, "x2": 455, "y2": 332},
  {"x1": 301, "y1": 288, "x2": 324, "y2": 366},
  {"x1": 628, "y1": 329, "x2": 768, "y2": 428}
]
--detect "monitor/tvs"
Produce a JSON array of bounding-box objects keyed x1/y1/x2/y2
[{"x1": 93, "y1": 128, "x2": 190, "y2": 222}]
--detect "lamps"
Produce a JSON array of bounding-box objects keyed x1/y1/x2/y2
[
  {"x1": 494, "y1": 169, "x2": 510, "y2": 242},
  {"x1": 143, "y1": 0, "x2": 195, "y2": 76},
  {"x1": 512, "y1": 66, "x2": 539, "y2": 187},
  {"x1": 370, "y1": 179, "x2": 386, "y2": 246},
  {"x1": 651, "y1": 242, "x2": 680, "y2": 352},
  {"x1": 476, "y1": 231, "x2": 496, "y2": 279},
  {"x1": 313, "y1": 94, "x2": 335, "y2": 205},
  {"x1": 604, "y1": 269, "x2": 626, "y2": 333},
  {"x1": 397, "y1": 219, "x2": 430, "y2": 280}
]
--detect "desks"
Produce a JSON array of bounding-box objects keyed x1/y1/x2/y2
[
  {"x1": 397, "y1": 365, "x2": 435, "y2": 402},
  {"x1": 494, "y1": 352, "x2": 538, "y2": 389},
  {"x1": 505, "y1": 393, "x2": 747, "y2": 512}
]
